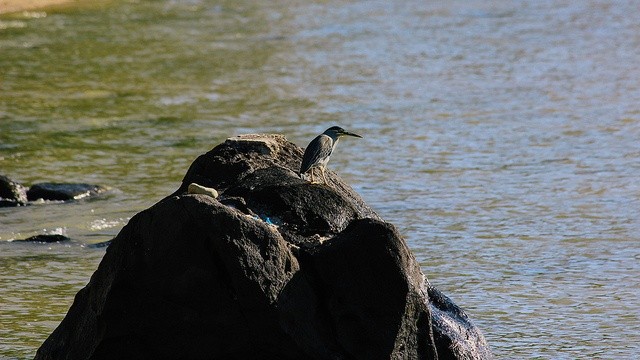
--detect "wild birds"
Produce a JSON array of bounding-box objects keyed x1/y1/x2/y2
[{"x1": 301, "y1": 126, "x2": 363, "y2": 189}]
